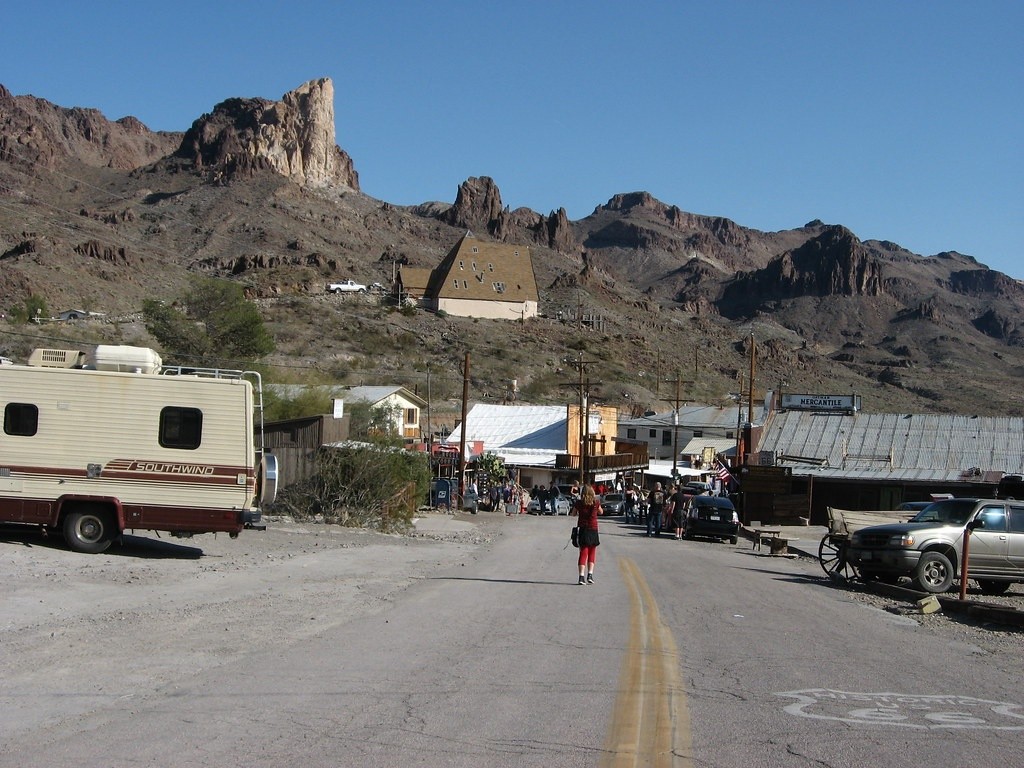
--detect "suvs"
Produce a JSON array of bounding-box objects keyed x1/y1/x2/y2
[{"x1": 845, "y1": 497, "x2": 1024, "y2": 595}]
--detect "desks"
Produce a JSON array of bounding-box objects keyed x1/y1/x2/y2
[
  {"x1": 760, "y1": 535, "x2": 799, "y2": 554},
  {"x1": 753, "y1": 527, "x2": 781, "y2": 551}
]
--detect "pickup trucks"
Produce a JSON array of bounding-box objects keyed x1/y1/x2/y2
[{"x1": 325, "y1": 280, "x2": 367, "y2": 294}]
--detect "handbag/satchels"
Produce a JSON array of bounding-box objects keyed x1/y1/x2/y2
[{"x1": 571, "y1": 527, "x2": 579, "y2": 547}]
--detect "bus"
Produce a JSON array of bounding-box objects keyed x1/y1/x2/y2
[{"x1": 0, "y1": 341, "x2": 280, "y2": 554}]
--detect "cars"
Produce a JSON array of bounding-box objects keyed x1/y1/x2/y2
[
  {"x1": 894, "y1": 502, "x2": 935, "y2": 512},
  {"x1": 680, "y1": 495, "x2": 739, "y2": 544},
  {"x1": 516, "y1": 483, "x2": 626, "y2": 516},
  {"x1": 464, "y1": 485, "x2": 480, "y2": 515},
  {"x1": 658, "y1": 480, "x2": 713, "y2": 532}
]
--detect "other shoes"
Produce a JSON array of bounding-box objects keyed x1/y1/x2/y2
[
  {"x1": 645, "y1": 533, "x2": 660, "y2": 538},
  {"x1": 673, "y1": 536, "x2": 683, "y2": 540}
]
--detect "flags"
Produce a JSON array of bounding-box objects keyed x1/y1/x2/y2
[{"x1": 714, "y1": 462, "x2": 730, "y2": 482}]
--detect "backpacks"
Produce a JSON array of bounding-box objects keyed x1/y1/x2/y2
[{"x1": 654, "y1": 492, "x2": 663, "y2": 505}]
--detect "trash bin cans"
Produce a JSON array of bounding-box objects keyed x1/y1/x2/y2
[{"x1": 435, "y1": 479, "x2": 451, "y2": 512}]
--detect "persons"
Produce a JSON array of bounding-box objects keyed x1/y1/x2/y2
[
  {"x1": 549, "y1": 481, "x2": 560, "y2": 516},
  {"x1": 625, "y1": 487, "x2": 638, "y2": 524},
  {"x1": 591, "y1": 479, "x2": 624, "y2": 494},
  {"x1": 490, "y1": 481, "x2": 525, "y2": 516},
  {"x1": 667, "y1": 484, "x2": 686, "y2": 540},
  {"x1": 572, "y1": 484, "x2": 603, "y2": 585},
  {"x1": 529, "y1": 485, "x2": 539, "y2": 500},
  {"x1": 538, "y1": 485, "x2": 546, "y2": 516},
  {"x1": 571, "y1": 480, "x2": 581, "y2": 516},
  {"x1": 709, "y1": 491, "x2": 713, "y2": 496},
  {"x1": 646, "y1": 482, "x2": 666, "y2": 537}
]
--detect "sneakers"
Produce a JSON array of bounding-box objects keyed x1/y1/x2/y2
[
  {"x1": 587, "y1": 572, "x2": 594, "y2": 584},
  {"x1": 578, "y1": 575, "x2": 586, "y2": 585}
]
